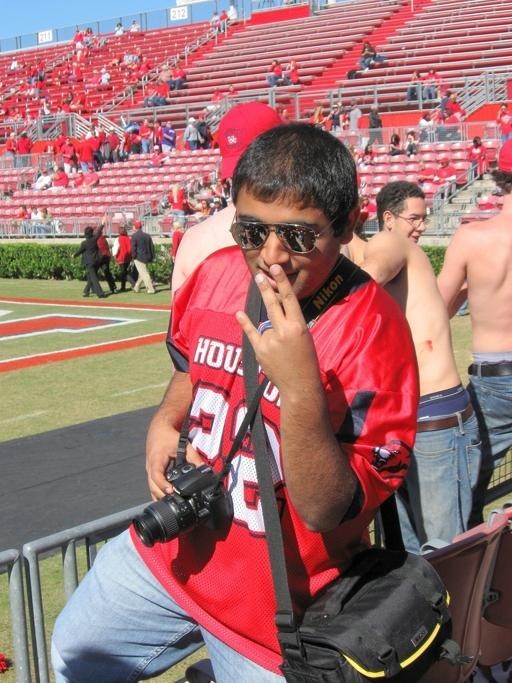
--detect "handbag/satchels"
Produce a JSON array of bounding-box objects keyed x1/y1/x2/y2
[{"x1": 281, "y1": 548, "x2": 452, "y2": 683}]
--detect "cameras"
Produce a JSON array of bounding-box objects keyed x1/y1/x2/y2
[{"x1": 132, "y1": 463, "x2": 233, "y2": 547}]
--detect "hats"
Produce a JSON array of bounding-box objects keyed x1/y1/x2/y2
[
  {"x1": 499, "y1": 138, "x2": 512, "y2": 173},
  {"x1": 219, "y1": 102, "x2": 283, "y2": 185}
]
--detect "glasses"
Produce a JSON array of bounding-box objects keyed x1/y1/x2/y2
[
  {"x1": 388, "y1": 209, "x2": 431, "y2": 226},
  {"x1": 228, "y1": 210, "x2": 338, "y2": 255}
]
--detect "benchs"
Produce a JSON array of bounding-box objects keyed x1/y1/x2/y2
[{"x1": 128, "y1": 1, "x2": 512, "y2": 118}]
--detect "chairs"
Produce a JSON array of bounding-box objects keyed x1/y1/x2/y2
[
  {"x1": 0, "y1": 130, "x2": 511, "y2": 240},
  {"x1": 410, "y1": 512, "x2": 505, "y2": 682},
  {"x1": 1, "y1": 1, "x2": 222, "y2": 131},
  {"x1": 480, "y1": 504, "x2": 512, "y2": 666}
]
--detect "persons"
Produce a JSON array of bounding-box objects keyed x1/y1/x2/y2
[
  {"x1": 339, "y1": 230, "x2": 483, "y2": 556},
  {"x1": 156, "y1": 165, "x2": 231, "y2": 238},
  {"x1": 432, "y1": 103, "x2": 512, "y2": 209},
  {"x1": 348, "y1": 130, "x2": 420, "y2": 166},
  {"x1": 376, "y1": 181, "x2": 426, "y2": 243},
  {"x1": 6, "y1": 204, "x2": 50, "y2": 239},
  {"x1": 347, "y1": 42, "x2": 376, "y2": 79},
  {"x1": 359, "y1": 181, "x2": 376, "y2": 219},
  {"x1": 210, "y1": 3, "x2": 238, "y2": 31},
  {"x1": 1, "y1": 20, "x2": 212, "y2": 190},
  {"x1": 418, "y1": 159, "x2": 435, "y2": 193},
  {"x1": 407, "y1": 69, "x2": 442, "y2": 100},
  {"x1": 268, "y1": 58, "x2": 298, "y2": 88},
  {"x1": 50, "y1": 119, "x2": 420, "y2": 683},
  {"x1": 309, "y1": 97, "x2": 383, "y2": 145},
  {"x1": 71, "y1": 209, "x2": 156, "y2": 297},
  {"x1": 172, "y1": 100, "x2": 287, "y2": 303},
  {"x1": 436, "y1": 137, "x2": 512, "y2": 531},
  {"x1": 417, "y1": 89, "x2": 466, "y2": 142}
]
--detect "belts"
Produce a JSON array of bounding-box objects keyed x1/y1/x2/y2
[
  {"x1": 416, "y1": 402, "x2": 474, "y2": 433},
  {"x1": 468, "y1": 362, "x2": 512, "y2": 376}
]
